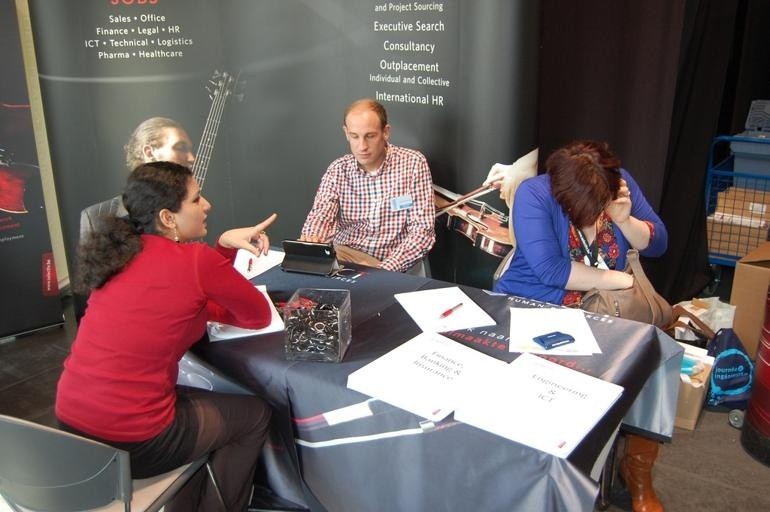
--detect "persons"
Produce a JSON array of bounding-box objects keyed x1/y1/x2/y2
[
  {"x1": 77, "y1": 116, "x2": 197, "y2": 245},
  {"x1": 51, "y1": 162, "x2": 278, "y2": 511},
  {"x1": 297, "y1": 98, "x2": 438, "y2": 280},
  {"x1": 483, "y1": 147, "x2": 539, "y2": 291},
  {"x1": 489, "y1": 136, "x2": 677, "y2": 512}
]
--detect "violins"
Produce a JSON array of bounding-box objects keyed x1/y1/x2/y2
[{"x1": 432, "y1": 186, "x2": 513, "y2": 259}]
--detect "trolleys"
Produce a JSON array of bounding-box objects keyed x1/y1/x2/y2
[{"x1": 696, "y1": 134, "x2": 769, "y2": 295}]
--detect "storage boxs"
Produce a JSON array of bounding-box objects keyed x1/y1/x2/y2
[{"x1": 674, "y1": 186, "x2": 770, "y2": 432}]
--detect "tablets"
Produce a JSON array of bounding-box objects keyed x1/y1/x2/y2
[{"x1": 280, "y1": 240, "x2": 339, "y2": 275}]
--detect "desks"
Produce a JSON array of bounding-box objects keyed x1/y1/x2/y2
[{"x1": 171, "y1": 241, "x2": 654, "y2": 510}]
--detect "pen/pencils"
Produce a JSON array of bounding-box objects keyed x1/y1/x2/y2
[
  {"x1": 248, "y1": 258, "x2": 253, "y2": 273},
  {"x1": 439, "y1": 303, "x2": 463, "y2": 319}
]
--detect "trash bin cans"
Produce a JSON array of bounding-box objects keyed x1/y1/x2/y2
[{"x1": 730, "y1": 99, "x2": 770, "y2": 192}]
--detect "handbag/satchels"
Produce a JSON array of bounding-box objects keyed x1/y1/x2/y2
[
  {"x1": 704, "y1": 327, "x2": 755, "y2": 413},
  {"x1": 581, "y1": 246, "x2": 677, "y2": 333}
]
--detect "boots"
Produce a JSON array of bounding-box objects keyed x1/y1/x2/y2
[{"x1": 615, "y1": 433, "x2": 666, "y2": 512}]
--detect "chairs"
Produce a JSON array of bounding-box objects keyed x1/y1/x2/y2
[{"x1": 0, "y1": 413, "x2": 255, "y2": 512}]
